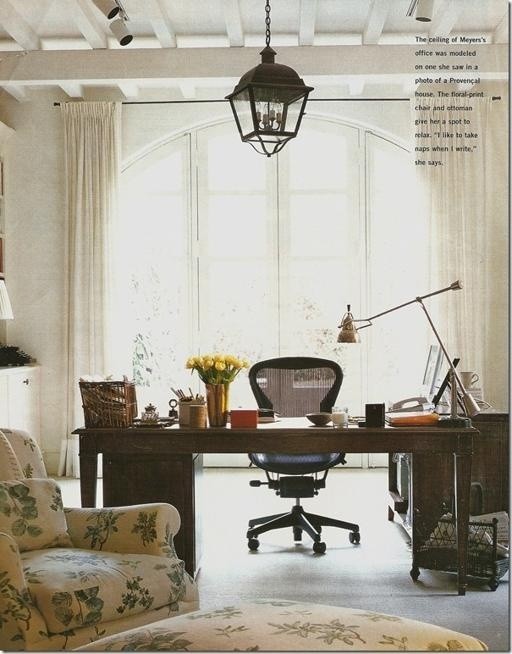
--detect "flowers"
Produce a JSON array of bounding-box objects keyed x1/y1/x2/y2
[{"x1": 187, "y1": 356, "x2": 248, "y2": 383}]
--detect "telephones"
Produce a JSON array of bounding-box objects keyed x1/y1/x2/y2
[{"x1": 389, "y1": 397, "x2": 435, "y2": 412}]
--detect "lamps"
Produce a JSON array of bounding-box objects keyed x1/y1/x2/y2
[
  {"x1": 225, "y1": 0, "x2": 314, "y2": 159},
  {"x1": 92, "y1": 0, "x2": 120, "y2": 20},
  {"x1": 110, "y1": 20, "x2": 133, "y2": 46},
  {"x1": 338, "y1": 279, "x2": 484, "y2": 416},
  {"x1": 416, "y1": 0, "x2": 435, "y2": 22},
  {"x1": 0, "y1": 276, "x2": 36, "y2": 366}
]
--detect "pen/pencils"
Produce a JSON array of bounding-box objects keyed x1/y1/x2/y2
[
  {"x1": 196, "y1": 394, "x2": 198, "y2": 400},
  {"x1": 171, "y1": 388, "x2": 186, "y2": 400},
  {"x1": 189, "y1": 388, "x2": 194, "y2": 399}
]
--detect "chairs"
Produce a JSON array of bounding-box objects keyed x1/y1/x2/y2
[{"x1": 246, "y1": 357, "x2": 361, "y2": 554}]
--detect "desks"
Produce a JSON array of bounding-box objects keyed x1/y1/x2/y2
[{"x1": 72, "y1": 412, "x2": 510, "y2": 596}]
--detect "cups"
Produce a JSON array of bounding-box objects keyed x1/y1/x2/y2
[
  {"x1": 460, "y1": 371, "x2": 480, "y2": 388},
  {"x1": 332, "y1": 407, "x2": 348, "y2": 428}
]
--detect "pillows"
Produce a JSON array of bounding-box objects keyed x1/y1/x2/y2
[{"x1": 0, "y1": 478, "x2": 72, "y2": 553}]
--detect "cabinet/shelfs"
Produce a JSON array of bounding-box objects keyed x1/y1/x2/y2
[{"x1": 0, "y1": 365, "x2": 42, "y2": 458}]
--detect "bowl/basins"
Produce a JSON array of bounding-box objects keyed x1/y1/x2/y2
[{"x1": 306, "y1": 414, "x2": 333, "y2": 425}]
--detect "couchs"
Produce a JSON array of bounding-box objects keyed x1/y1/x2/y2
[
  {"x1": 70, "y1": 599, "x2": 489, "y2": 654},
  {"x1": 1, "y1": 428, "x2": 200, "y2": 652}
]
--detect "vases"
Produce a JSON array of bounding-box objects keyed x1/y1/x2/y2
[{"x1": 205, "y1": 382, "x2": 230, "y2": 426}]
